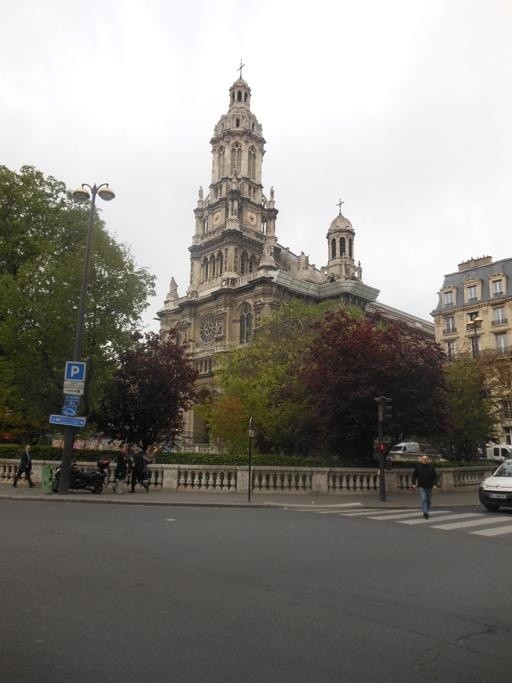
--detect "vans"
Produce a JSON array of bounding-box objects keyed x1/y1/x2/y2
[
  {"x1": 390, "y1": 440, "x2": 420, "y2": 454},
  {"x1": 478, "y1": 458, "x2": 512, "y2": 511}
]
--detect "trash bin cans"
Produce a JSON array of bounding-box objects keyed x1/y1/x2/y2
[{"x1": 40, "y1": 465, "x2": 56, "y2": 495}]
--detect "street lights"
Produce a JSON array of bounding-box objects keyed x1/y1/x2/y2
[
  {"x1": 467, "y1": 316, "x2": 483, "y2": 357},
  {"x1": 54, "y1": 181, "x2": 117, "y2": 491}
]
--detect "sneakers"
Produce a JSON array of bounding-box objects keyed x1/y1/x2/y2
[{"x1": 424, "y1": 513, "x2": 430, "y2": 520}]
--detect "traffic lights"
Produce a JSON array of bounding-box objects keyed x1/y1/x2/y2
[
  {"x1": 380, "y1": 396, "x2": 392, "y2": 419},
  {"x1": 376, "y1": 441, "x2": 385, "y2": 453}
]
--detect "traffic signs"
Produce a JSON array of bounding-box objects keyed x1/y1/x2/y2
[
  {"x1": 50, "y1": 414, "x2": 87, "y2": 427},
  {"x1": 63, "y1": 380, "x2": 85, "y2": 395}
]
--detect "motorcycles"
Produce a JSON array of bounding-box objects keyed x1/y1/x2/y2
[{"x1": 49, "y1": 458, "x2": 110, "y2": 494}]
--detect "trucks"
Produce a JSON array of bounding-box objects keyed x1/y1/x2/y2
[{"x1": 486, "y1": 444, "x2": 512, "y2": 463}]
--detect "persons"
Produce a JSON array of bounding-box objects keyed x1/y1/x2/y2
[
  {"x1": 412, "y1": 455, "x2": 439, "y2": 519},
  {"x1": 13, "y1": 444, "x2": 37, "y2": 488},
  {"x1": 112, "y1": 444, "x2": 128, "y2": 493},
  {"x1": 128, "y1": 445, "x2": 149, "y2": 493}
]
